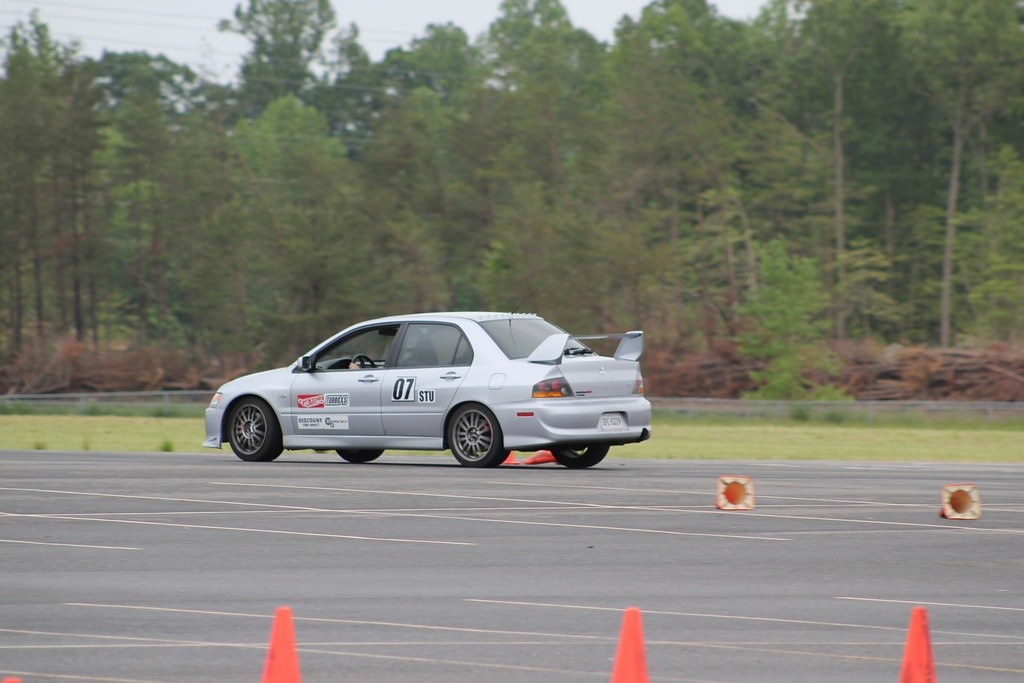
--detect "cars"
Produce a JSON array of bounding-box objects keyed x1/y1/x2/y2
[{"x1": 203, "y1": 311, "x2": 651, "y2": 468}]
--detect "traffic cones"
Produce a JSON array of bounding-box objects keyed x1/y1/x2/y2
[
  {"x1": 258, "y1": 606, "x2": 302, "y2": 683},
  {"x1": 523, "y1": 449, "x2": 556, "y2": 465},
  {"x1": 716, "y1": 474, "x2": 755, "y2": 510},
  {"x1": 607, "y1": 607, "x2": 651, "y2": 683},
  {"x1": 939, "y1": 481, "x2": 982, "y2": 520},
  {"x1": 503, "y1": 449, "x2": 520, "y2": 466},
  {"x1": 897, "y1": 605, "x2": 939, "y2": 683}
]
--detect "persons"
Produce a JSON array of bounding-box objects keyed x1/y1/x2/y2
[{"x1": 348, "y1": 325, "x2": 424, "y2": 369}]
